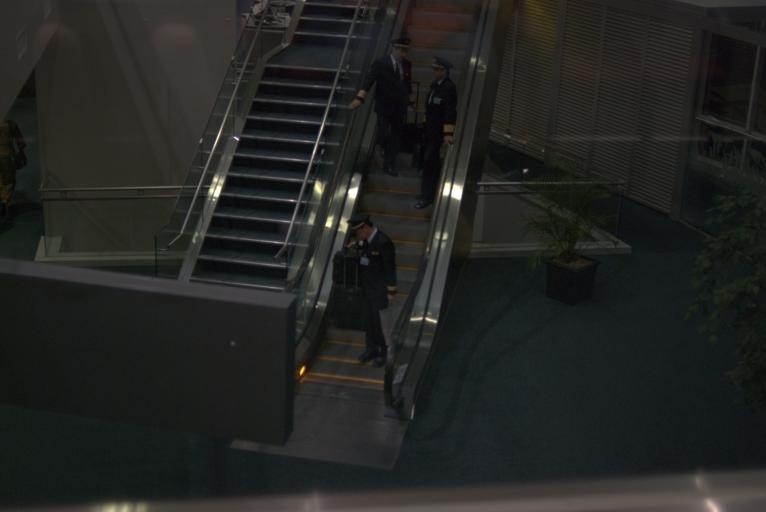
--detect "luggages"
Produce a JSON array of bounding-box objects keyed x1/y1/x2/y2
[
  {"x1": 402, "y1": 81, "x2": 423, "y2": 154},
  {"x1": 335, "y1": 255, "x2": 368, "y2": 330}
]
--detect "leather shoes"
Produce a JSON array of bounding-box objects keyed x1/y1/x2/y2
[
  {"x1": 383, "y1": 165, "x2": 398, "y2": 177},
  {"x1": 358, "y1": 349, "x2": 375, "y2": 362},
  {"x1": 414, "y1": 198, "x2": 431, "y2": 209},
  {"x1": 373, "y1": 354, "x2": 386, "y2": 368}
]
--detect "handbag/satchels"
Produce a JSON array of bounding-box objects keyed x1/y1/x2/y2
[
  {"x1": 413, "y1": 144, "x2": 425, "y2": 169},
  {"x1": 333, "y1": 251, "x2": 368, "y2": 285}
]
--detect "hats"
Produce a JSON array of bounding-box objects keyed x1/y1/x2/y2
[
  {"x1": 347, "y1": 215, "x2": 371, "y2": 231},
  {"x1": 431, "y1": 56, "x2": 453, "y2": 69},
  {"x1": 390, "y1": 38, "x2": 410, "y2": 53}
]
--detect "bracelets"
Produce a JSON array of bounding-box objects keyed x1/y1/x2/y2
[{"x1": 354, "y1": 96, "x2": 365, "y2": 104}]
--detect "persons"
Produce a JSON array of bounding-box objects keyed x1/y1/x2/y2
[
  {"x1": 345, "y1": 213, "x2": 397, "y2": 368},
  {"x1": 414, "y1": 55, "x2": 459, "y2": 211},
  {"x1": 0, "y1": 119, "x2": 28, "y2": 230},
  {"x1": 349, "y1": 36, "x2": 413, "y2": 176}
]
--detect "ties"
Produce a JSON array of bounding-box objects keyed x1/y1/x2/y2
[{"x1": 396, "y1": 63, "x2": 400, "y2": 81}]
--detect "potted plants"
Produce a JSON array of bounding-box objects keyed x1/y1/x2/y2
[{"x1": 517, "y1": 142, "x2": 622, "y2": 304}]
